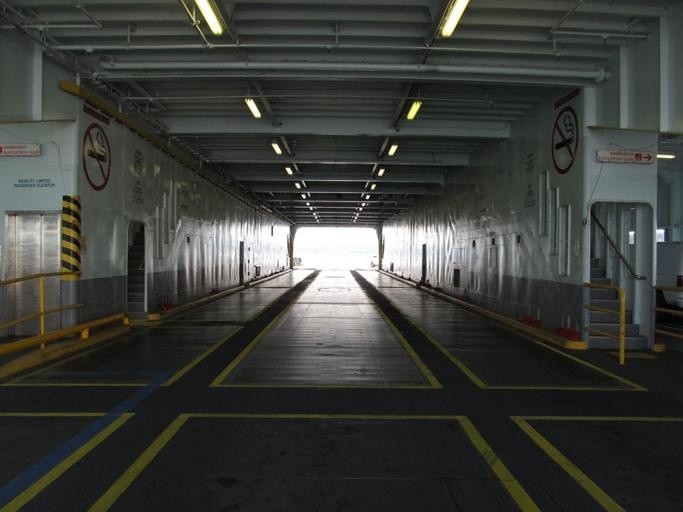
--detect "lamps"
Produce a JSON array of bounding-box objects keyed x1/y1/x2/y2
[
  {"x1": 285, "y1": 163, "x2": 300, "y2": 179},
  {"x1": 657, "y1": 150, "x2": 676, "y2": 159},
  {"x1": 270, "y1": 136, "x2": 291, "y2": 156},
  {"x1": 370, "y1": 164, "x2": 386, "y2": 177},
  {"x1": 364, "y1": 183, "x2": 376, "y2": 191},
  {"x1": 378, "y1": 136, "x2": 399, "y2": 159},
  {"x1": 427, "y1": 0, "x2": 470, "y2": 47},
  {"x1": 245, "y1": 81, "x2": 272, "y2": 119},
  {"x1": 391, "y1": 81, "x2": 424, "y2": 129},
  {"x1": 293, "y1": 180, "x2": 306, "y2": 191},
  {"x1": 179, "y1": 0, "x2": 239, "y2": 48}
]
